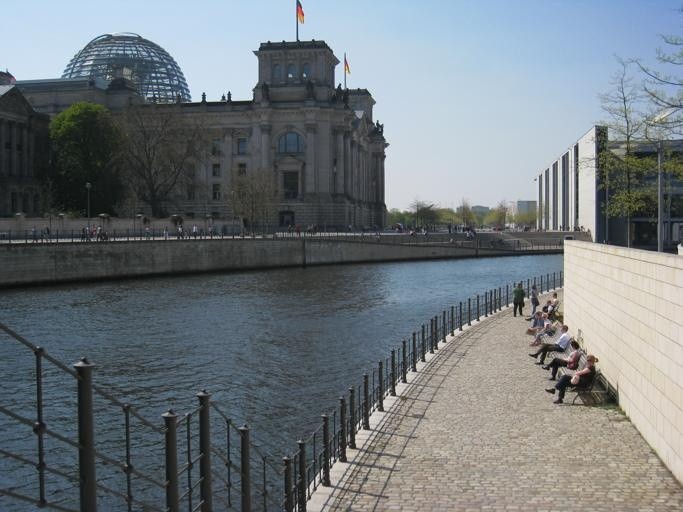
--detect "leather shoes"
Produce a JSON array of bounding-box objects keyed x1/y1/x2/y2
[
  {"x1": 529, "y1": 353, "x2": 550, "y2": 370},
  {"x1": 549, "y1": 377, "x2": 555, "y2": 380}
]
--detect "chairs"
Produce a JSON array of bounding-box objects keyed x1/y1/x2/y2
[{"x1": 536, "y1": 297, "x2": 610, "y2": 407}]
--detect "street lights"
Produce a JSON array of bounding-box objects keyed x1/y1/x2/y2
[{"x1": 85, "y1": 182, "x2": 92, "y2": 226}]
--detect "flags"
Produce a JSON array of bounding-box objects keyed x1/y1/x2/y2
[
  {"x1": 296, "y1": 0, "x2": 305, "y2": 25},
  {"x1": 344, "y1": 55, "x2": 351, "y2": 76}
]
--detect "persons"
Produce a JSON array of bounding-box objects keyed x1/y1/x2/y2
[
  {"x1": 96, "y1": 225, "x2": 104, "y2": 241},
  {"x1": 192, "y1": 224, "x2": 197, "y2": 239},
  {"x1": 287, "y1": 224, "x2": 318, "y2": 236},
  {"x1": 526, "y1": 300, "x2": 553, "y2": 328},
  {"x1": 526, "y1": 311, "x2": 544, "y2": 337},
  {"x1": 529, "y1": 325, "x2": 570, "y2": 365},
  {"x1": 145, "y1": 221, "x2": 151, "y2": 240},
  {"x1": 530, "y1": 285, "x2": 539, "y2": 315},
  {"x1": 80, "y1": 224, "x2": 85, "y2": 242},
  {"x1": 542, "y1": 292, "x2": 558, "y2": 311},
  {"x1": 31, "y1": 223, "x2": 39, "y2": 243},
  {"x1": 44, "y1": 224, "x2": 50, "y2": 243},
  {"x1": 545, "y1": 354, "x2": 598, "y2": 404},
  {"x1": 542, "y1": 341, "x2": 580, "y2": 380},
  {"x1": 176, "y1": 224, "x2": 185, "y2": 240},
  {"x1": 511, "y1": 282, "x2": 525, "y2": 317},
  {"x1": 529, "y1": 312, "x2": 553, "y2": 345}
]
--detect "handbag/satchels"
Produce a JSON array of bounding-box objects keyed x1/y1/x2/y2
[
  {"x1": 571, "y1": 375, "x2": 580, "y2": 385},
  {"x1": 567, "y1": 362, "x2": 577, "y2": 368}
]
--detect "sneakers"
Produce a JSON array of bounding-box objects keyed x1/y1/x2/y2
[
  {"x1": 545, "y1": 388, "x2": 555, "y2": 394},
  {"x1": 553, "y1": 399, "x2": 563, "y2": 404}
]
